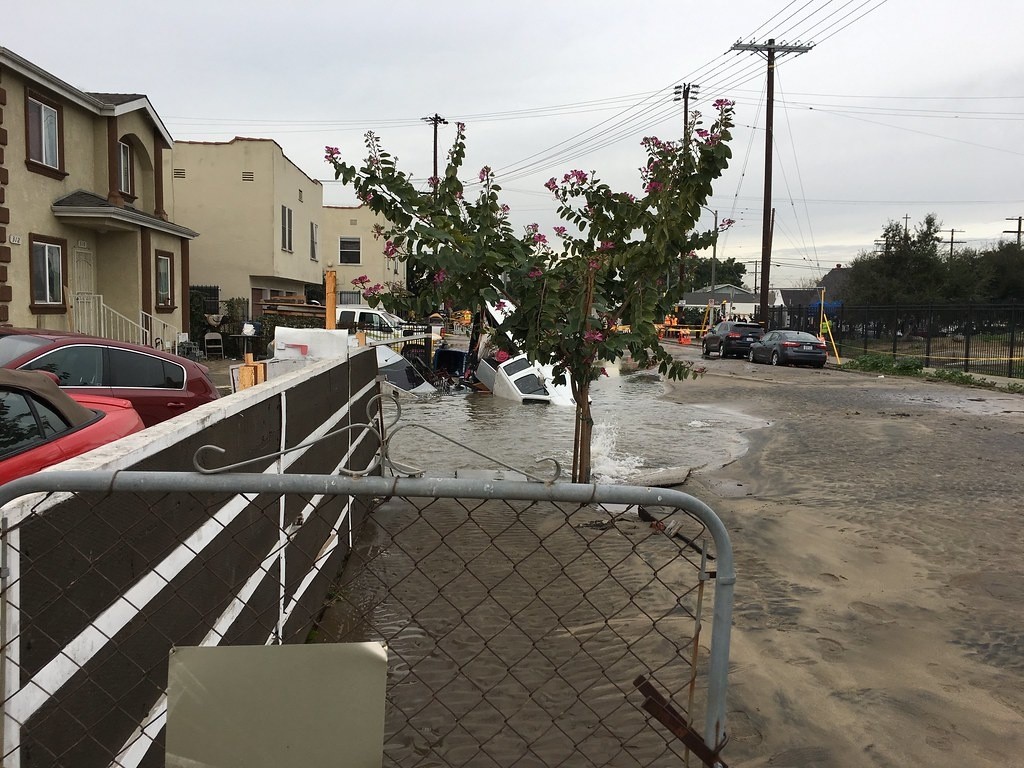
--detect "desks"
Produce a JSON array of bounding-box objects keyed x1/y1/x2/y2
[{"x1": 230, "y1": 334, "x2": 260, "y2": 364}]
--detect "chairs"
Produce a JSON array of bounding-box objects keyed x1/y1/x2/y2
[{"x1": 204, "y1": 332, "x2": 224, "y2": 360}]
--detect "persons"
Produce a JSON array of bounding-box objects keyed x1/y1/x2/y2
[
  {"x1": 821, "y1": 316, "x2": 833, "y2": 341},
  {"x1": 701, "y1": 314, "x2": 756, "y2": 325}
]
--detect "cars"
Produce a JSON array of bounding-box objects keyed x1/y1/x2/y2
[
  {"x1": 335, "y1": 304, "x2": 445, "y2": 349},
  {"x1": 1, "y1": 365, "x2": 150, "y2": 493},
  {"x1": 702, "y1": 320, "x2": 765, "y2": 359},
  {"x1": 749, "y1": 329, "x2": 828, "y2": 369},
  {"x1": 1, "y1": 324, "x2": 222, "y2": 428},
  {"x1": 264, "y1": 332, "x2": 436, "y2": 400},
  {"x1": 844, "y1": 319, "x2": 1016, "y2": 338}
]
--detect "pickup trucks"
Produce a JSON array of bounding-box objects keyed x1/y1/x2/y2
[{"x1": 475, "y1": 292, "x2": 593, "y2": 410}]
